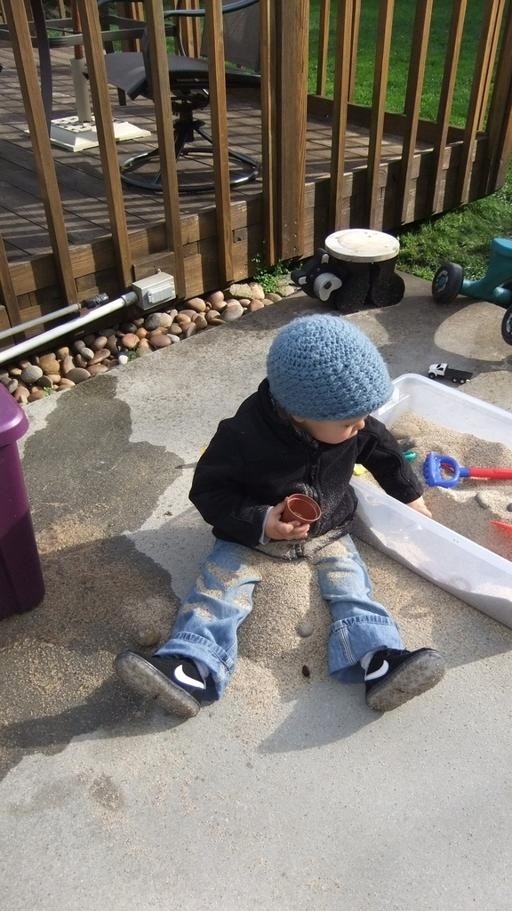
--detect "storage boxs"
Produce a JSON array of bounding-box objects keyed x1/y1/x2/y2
[{"x1": 348, "y1": 371, "x2": 511, "y2": 630}]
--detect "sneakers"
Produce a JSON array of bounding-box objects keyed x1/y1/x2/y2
[
  {"x1": 365, "y1": 648, "x2": 447, "y2": 711},
  {"x1": 115, "y1": 649, "x2": 206, "y2": 718}
]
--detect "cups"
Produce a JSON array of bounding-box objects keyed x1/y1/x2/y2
[{"x1": 281, "y1": 491, "x2": 322, "y2": 529}]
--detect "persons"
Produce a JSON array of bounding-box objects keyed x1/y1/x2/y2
[{"x1": 115, "y1": 314, "x2": 447, "y2": 719}]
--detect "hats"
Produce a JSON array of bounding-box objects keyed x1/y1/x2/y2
[{"x1": 267, "y1": 313, "x2": 395, "y2": 421}]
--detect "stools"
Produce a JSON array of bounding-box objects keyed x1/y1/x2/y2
[{"x1": 290, "y1": 228, "x2": 407, "y2": 316}]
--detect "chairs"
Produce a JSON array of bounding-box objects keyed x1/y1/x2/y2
[{"x1": 79, "y1": 0, "x2": 262, "y2": 198}]
